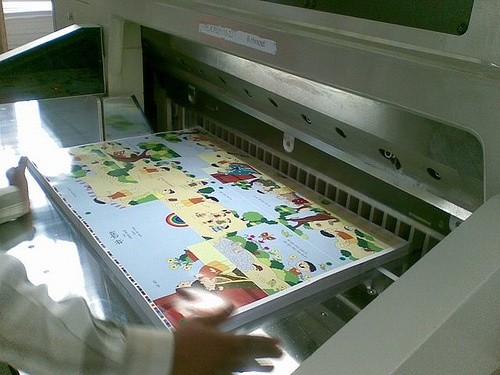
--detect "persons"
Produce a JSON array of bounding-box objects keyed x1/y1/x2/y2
[{"x1": 0, "y1": 155, "x2": 284, "y2": 375}]
